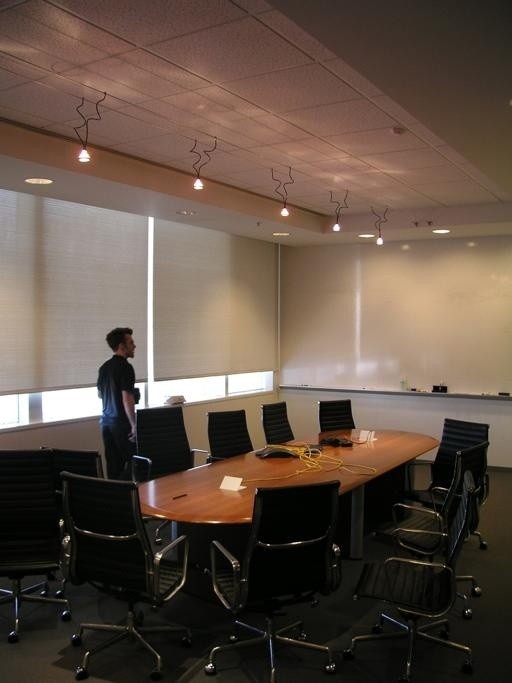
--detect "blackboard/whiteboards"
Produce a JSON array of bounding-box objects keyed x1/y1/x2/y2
[{"x1": 278, "y1": 236, "x2": 512, "y2": 401}]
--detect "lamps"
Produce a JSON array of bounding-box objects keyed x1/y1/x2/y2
[
  {"x1": 329, "y1": 189, "x2": 349, "y2": 232},
  {"x1": 272, "y1": 167, "x2": 294, "y2": 218},
  {"x1": 73, "y1": 90, "x2": 107, "y2": 163},
  {"x1": 190, "y1": 136, "x2": 216, "y2": 191},
  {"x1": 370, "y1": 206, "x2": 388, "y2": 246}
]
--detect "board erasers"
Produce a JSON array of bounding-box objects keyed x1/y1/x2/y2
[
  {"x1": 498, "y1": 393, "x2": 509, "y2": 396},
  {"x1": 433, "y1": 386, "x2": 447, "y2": 392}
]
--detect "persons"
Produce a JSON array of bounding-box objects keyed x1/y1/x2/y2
[{"x1": 97, "y1": 328, "x2": 137, "y2": 480}]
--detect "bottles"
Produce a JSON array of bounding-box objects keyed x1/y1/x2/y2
[
  {"x1": 410, "y1": 384, "x2": 416, "y2": 391},
  {"x1": 399, "y1": 375, "x2": 407, "y2": 392}
]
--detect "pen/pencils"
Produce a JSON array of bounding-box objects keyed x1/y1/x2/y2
[{"x1": 174, "y1": 494, "x2": 186, "y2": 499}]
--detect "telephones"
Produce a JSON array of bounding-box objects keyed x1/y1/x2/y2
[{"x1": 256, "y1": 448, "x2": 295, "y2": 458}]
